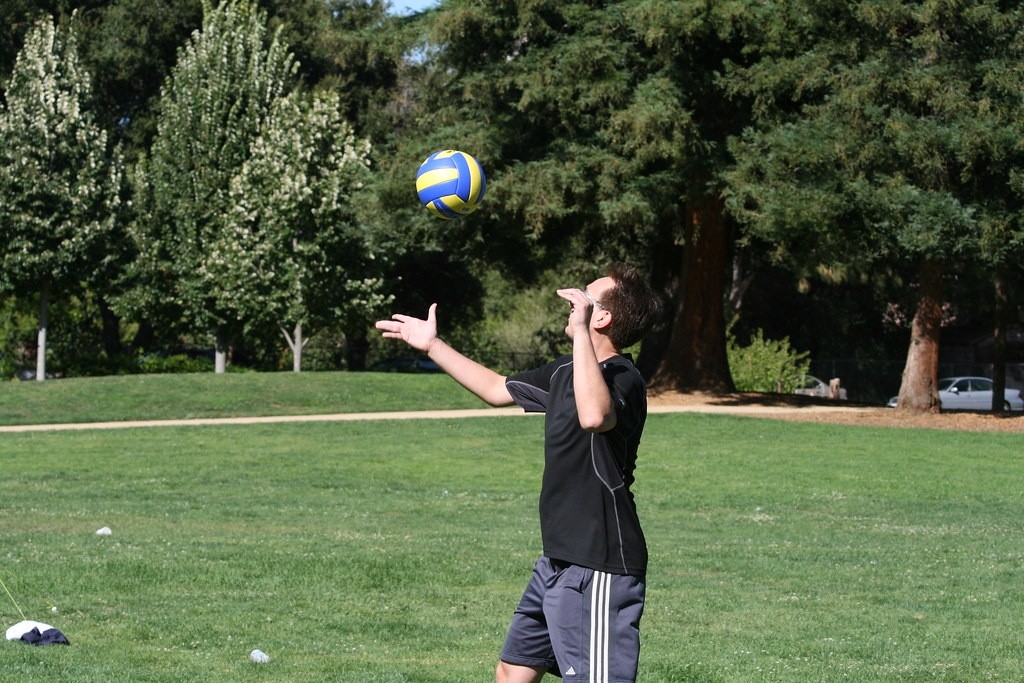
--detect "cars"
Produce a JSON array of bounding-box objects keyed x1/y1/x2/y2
[
  {"x1": 885, "y1": 376, "x2": 1024, "y2": 413},
  {"x1": 758, "y1": 375, "x2": 848, "y2": 401}
]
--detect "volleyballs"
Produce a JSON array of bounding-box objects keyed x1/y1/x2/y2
[{"x1": 413, "y1": 148, "x2": 487, "y2": 221}]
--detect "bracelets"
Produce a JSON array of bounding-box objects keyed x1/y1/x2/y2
[{"x1": 426, "y1": 338, "x2": 439, "y2": 353}]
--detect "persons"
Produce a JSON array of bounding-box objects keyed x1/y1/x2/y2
[{"x1": 374, "y1": 262, "x2": 666, "y2": 683}]
